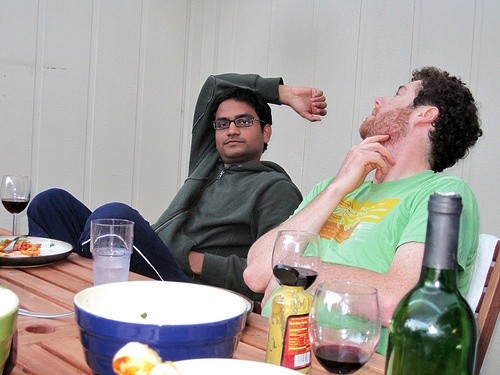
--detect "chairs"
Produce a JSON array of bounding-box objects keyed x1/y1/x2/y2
[{"x1": 466, "y1": 231, "x2": 500, "y2": 375}]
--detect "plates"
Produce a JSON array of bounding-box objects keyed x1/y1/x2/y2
[{"x1": 0, "y1": 237, "x2": 74, "y2": 268}]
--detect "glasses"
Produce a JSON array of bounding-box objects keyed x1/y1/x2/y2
[{"x1": 213, "y1": 116, "x2": 265, "y2": 130}]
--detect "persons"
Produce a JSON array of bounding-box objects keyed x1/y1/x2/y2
[
  {"x1": 242, "y1": 66, "x2": 484, "y2": 355},
  {"x1": 26, "y1": 73, "x2": 327, "y2": 297}
]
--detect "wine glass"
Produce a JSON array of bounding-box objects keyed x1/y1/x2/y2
[{"x1": 0, "y1": 175, "x2": 31, "y2": 236}]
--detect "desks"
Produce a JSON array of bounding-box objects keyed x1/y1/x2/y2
[{"x1": 0, "y1": 227, "x2": 389, "y2": 375}]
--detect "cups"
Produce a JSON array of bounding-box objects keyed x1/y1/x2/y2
[
  {"x1": 307, "y1": 282, "x2": 381, "y2": 375},
  {"x1": 90, "y1": 218, "x2": 134, "y2": 286},
  {"x1": 272, "y1": 230, "x2": 321, "y2": 290}
]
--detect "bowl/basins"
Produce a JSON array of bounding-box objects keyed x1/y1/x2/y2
[
  {"x1": 73, "y1": 280, "x2": 247, "y2": 375},
  {"x1": 0, "y1": 286, "x2": 20, "y2": 375},
  {"x1": 151, "y1": 357, "x2": 305, "y2": 375}
]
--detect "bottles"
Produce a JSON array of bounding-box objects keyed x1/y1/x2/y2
[
  {"x1": 384, "y1": 191, "x2": 477, "y2": 374},
  {"x1": 265, "y1": 265, "x2": 312, "y2": 375}
]
